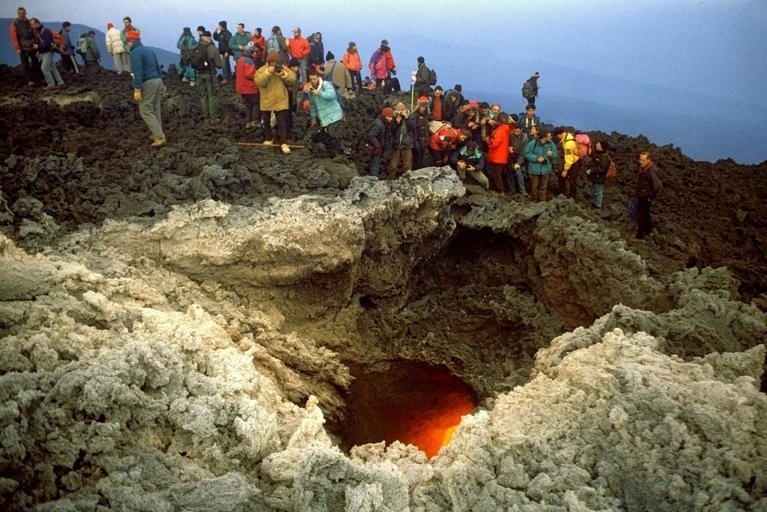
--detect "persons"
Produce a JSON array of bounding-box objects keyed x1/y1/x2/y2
[
  {"x1": 362, "y1": 40, "x2": 431, "y2": 93},
  {"x1": 627, "y1": 152, "x2": 662, "y2": 242},
  {"x1": 105, "y1": 21, "x2": 125, "y2": 75},
  {"x1": 9, "y1": 7, "x2": 101, "y2": 91},
  {"x1": 363, "y1": 84, "x2": 530, "y2": 198},
  {"x1": 126, "y1": 30, "x2": 167, "y2": 147},
  {"x1": 179, "y1": 21, "x2": 361, "y2": 159},
  {"x1": 519, "y1": 105, "x2": 610, "y2": 209},
  {"x1": 527, "y1": 72, "x2": 540, "y2": 104},
  {"x1": 120, "y1": 17, "x2": 141, "y2": 56}
]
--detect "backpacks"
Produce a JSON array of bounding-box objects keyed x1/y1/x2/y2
[
  {"x1": 565, "y1": 131, "x2": 591, "y2": 157},
  {"x1": 267, "y1": 35, "x2": 286, "y2": 56},
  {"x1": 428, "y1": 120, "x2": 456, "y2": 142},
  {"x1": 75, "y1": 36, "x2": 91, "y2": 52},
  {"x1": 191, "y1": 43, "x2": 215, "y2": 71}
]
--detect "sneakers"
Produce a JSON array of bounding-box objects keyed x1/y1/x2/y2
[
  {"x1": 204, "y1": 119, "x2": 220, "y2": 125},
  {"x1": 245, "y1": 121, "x2": 292, "y2": 155},
  {"x1": 177, "y1": 73, "x2": 196, "y2": 87},
  {"x1": 150, "y1": 135, "x2": 167, "y2": 146},
  {"x1": 43, "y1": 80, "x2": 63, "y2": 89}
]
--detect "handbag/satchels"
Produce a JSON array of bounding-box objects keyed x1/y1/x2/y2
[
  {"x1": 53, "y1": 30, "x2": 66, "y2": 53},
  {"x1": 521, "y1": 81, "x2": 534, "y2": 99},
  {"x1": 627, "y1": 197, "x2": 640, "y2": 216},
  {"x1": 182, "y1": 41, "x2": 192, "y2": 61},
  {"x1": 607, "y1": 164, "x2": 616, "y2": 177},
  {"x1": 347, "y1": 127, "x2": 374, "y2": 169}
]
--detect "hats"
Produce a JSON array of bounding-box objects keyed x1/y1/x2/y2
[
  {"x1": 380, "y1": 96, "x2": 433, "y2": 119},
  {"x1": 461, "y1": 101, "x2": 479, "y2": 117},
  {"x1": 292, "y1": 27, "x2": 300, "y2": 32},
  {"x1": 267, "y1": 50, "x2": 280, "y2": 61},
  {"x1": 125, "y1": 30, "x2": 140, "y2": 39},
  {"x1": 243, "y1": 44, "x2": 258, "y2": 55}
]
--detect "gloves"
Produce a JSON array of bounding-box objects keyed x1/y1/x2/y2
[{"x1": 134, "y1": 86, "x2": 142, "y2": 101}]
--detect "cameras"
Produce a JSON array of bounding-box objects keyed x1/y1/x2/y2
[{"x1": 273, "y1": 63, "x2": 281, "y2": 73}]
